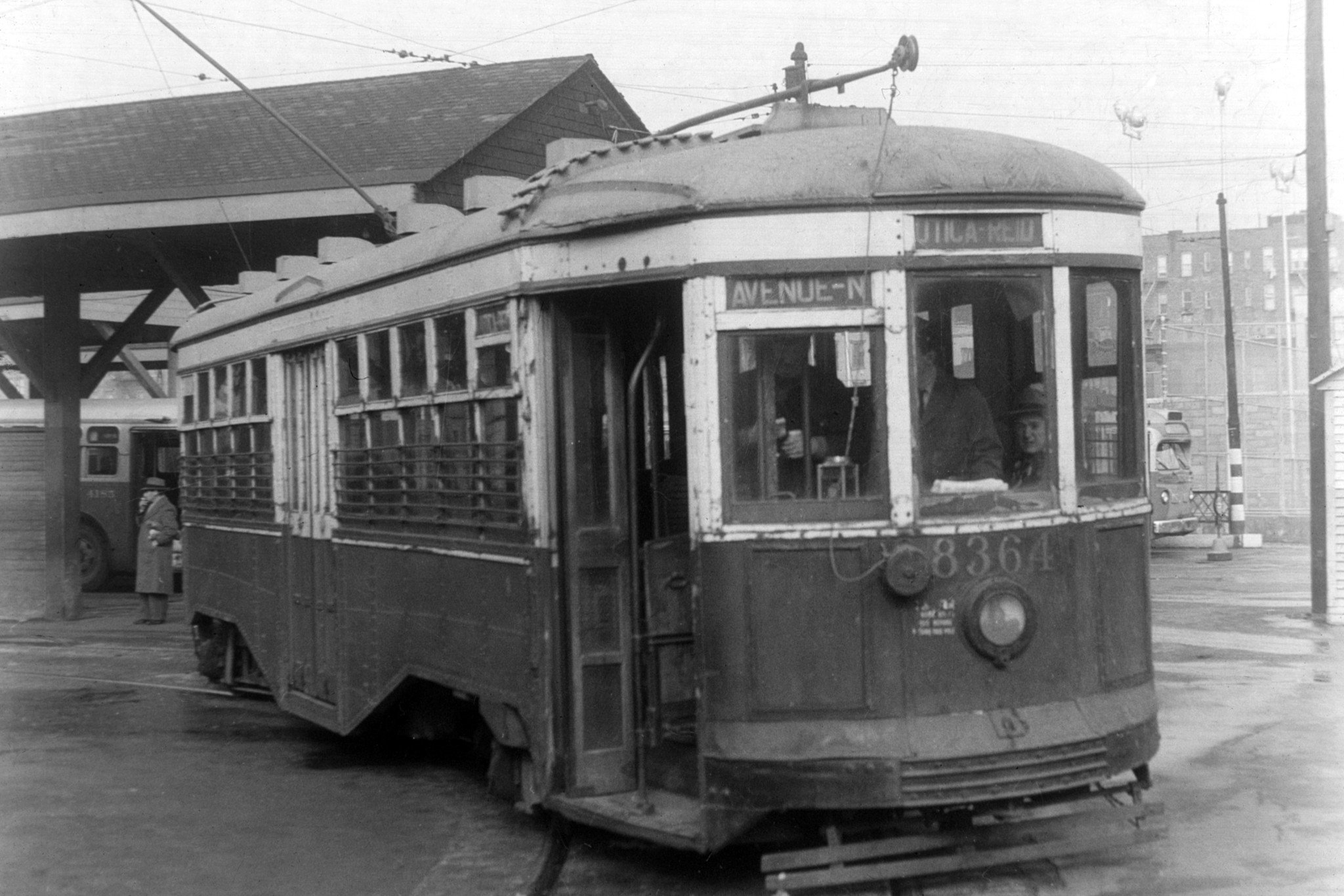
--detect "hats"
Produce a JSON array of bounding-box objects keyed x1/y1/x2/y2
[
  {"x1": 1004, "y1": 388, "x2": 1047, "y2": 417},
  {"x1": 140, "y1": 477, "x2": 171, "y2": 492},
  {"x1": 914, "y1": 313, "x2": 938, "y2": 347}
]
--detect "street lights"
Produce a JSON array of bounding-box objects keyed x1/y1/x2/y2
[
  {"x1": 1269, "y1": 156, "x2": 1298, "y2": 491},
  {"x1": 1214, "y1": 73, "x2": 1235, "y2": 197},
  {"x1": 1115, "y1": 101, "x2": 1145, "y2": 193}
]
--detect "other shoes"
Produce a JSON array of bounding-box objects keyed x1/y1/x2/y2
[
  {"x1": 134, "y1": 618, "x2": 150, "y2": 624},
  {"x1": 145, "y1": 619, "x2": 165, "y2": 625}
]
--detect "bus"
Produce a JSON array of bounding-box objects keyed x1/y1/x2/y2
[
  {"x1": 171, "y1": 31, "x2": 1165, "y2": 896},
  {"x1": 73, "y1": 419, "x2": 185, "y2": 595},
  {"x1": 1094, "y1": 406, "x2": 1200, "y2": 545}
]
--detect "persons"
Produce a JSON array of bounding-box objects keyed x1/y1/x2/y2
[
  {"x1": 913, "y1": 317, "x2": 1004, "y2": 483},
  {"x1": 997, "y1": 389, "x2": 1050, "y2": 489},
  {"x1": 133, "y1": 477, "x2": 178, "y2": 626},
  {"x1": 732, "y1": 335, "x2": 861, "y2": 498}
]
